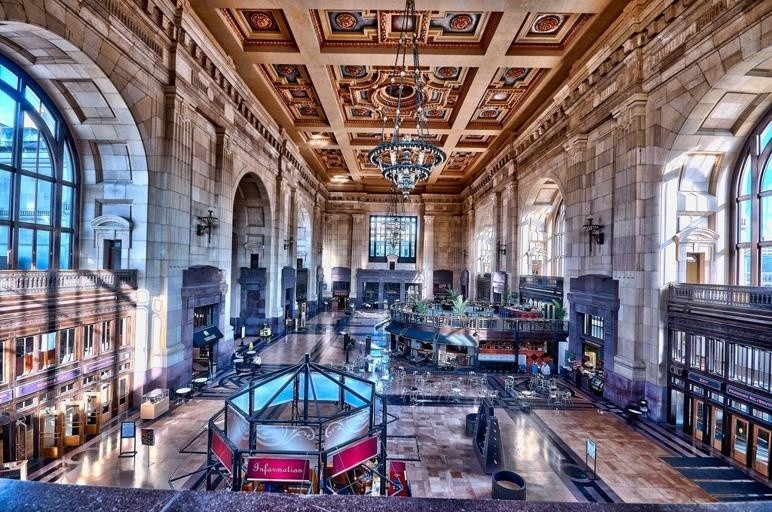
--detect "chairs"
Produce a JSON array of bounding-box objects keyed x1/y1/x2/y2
[{"x1": 331, "y1": 356, "x2": 574, "y2": 413}]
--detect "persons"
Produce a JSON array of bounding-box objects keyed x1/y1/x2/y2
[
  {"x1": 541, "y1": 360, "x2": 551, "y2": 380},
  {"x1": 253, "y1": 354, "x2": 265, "y2": 375},
  {"x1": 530, "y1": 360, "x2": 538, "y2": 374},
  {"x1": 548, "y1": 359, "x2": 556, "y2": 376},
  {"x1": 364, "y1": 352, "x2": 373, "y2": 373}
]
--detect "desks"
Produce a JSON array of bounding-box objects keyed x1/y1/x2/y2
[
  {"x1": 247, "y1": 350, "x2": 256, "y2": 355},
  {"x1": 176, "y1": 387, "x2": 191, "y2": 404},
  {"x1": 194, "y1": 377, "x2": 208, "y2": 390},
  {"x1": 233, "y1": 358, "x2": 244, "y2": 363}
]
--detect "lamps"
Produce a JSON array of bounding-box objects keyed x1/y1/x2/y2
[
  {"x1": 200, "y1": 205, "x2": 218, "y2": 244},
  {"x1": 582, "y1": 214, "x2": 602, "y2": 255},
  {"x1": 383, "y1": 183, "x2": 412, "y2": 248},
  {"x1": 368, "y1": 0, "x2": 449, "y2": 199}
]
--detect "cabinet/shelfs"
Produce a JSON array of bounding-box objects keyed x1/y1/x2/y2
[{"x1": 140, "y1": 388, "x2": 169, "y2": 421}]
